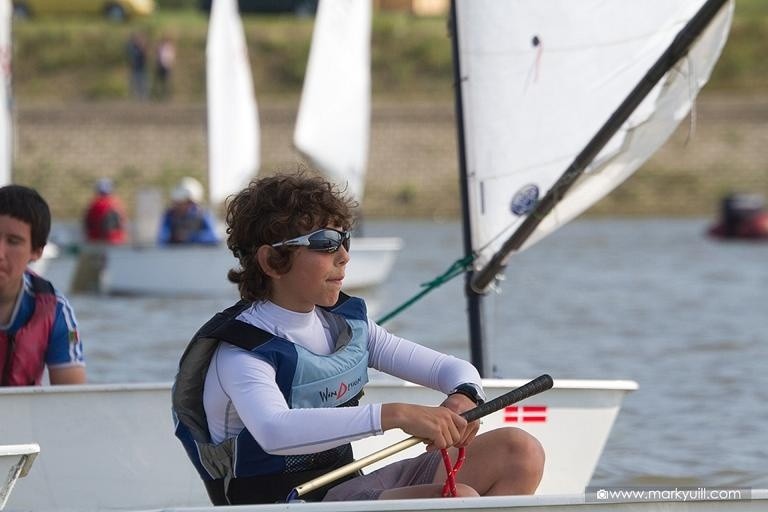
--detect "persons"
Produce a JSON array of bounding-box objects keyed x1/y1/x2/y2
[
  {"x1": 158, "y1": 177, "x2": 223, "y2": 247},
  {"x1": 84, "y1": 176, "x2": 129, "y2": 244},
  {"x1": 167, "y1": 174, "x2": 547, "y2": 502},
  {"x1": 127, "y1": 30, "x2": 150, "y2": 101},
  {"x1": 152, "y1": 31, "x2": 177, "y2": 103},
  {"x1": 0, "y1": 185, "x2": 86, "y2": 386}
]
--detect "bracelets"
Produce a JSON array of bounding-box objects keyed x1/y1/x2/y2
[{"x1": 448, "y1": 386, "x2": 485, "y2": 406}]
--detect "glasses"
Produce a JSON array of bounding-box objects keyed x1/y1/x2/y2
[{"x1": 251, "y1": 227, "x2": 351, "y2": 265}]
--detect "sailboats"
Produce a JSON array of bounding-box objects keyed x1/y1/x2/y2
[
  {"x1": 66, "y1": 0, "x2": 406, "y2": 306},
  {"x1": 0, "y1": 0, "x2": 737, "y2": 511}
]
